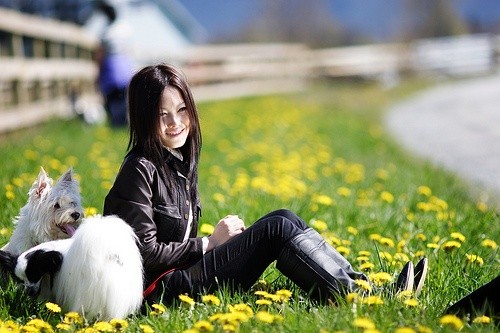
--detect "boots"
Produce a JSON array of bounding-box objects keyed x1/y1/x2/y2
[
  {"x1": 275, "y1": 234, "x2": 414, "y2": 310},
  {"x1": 302, "y1": 227, "x2": 428, "y2": 300}
]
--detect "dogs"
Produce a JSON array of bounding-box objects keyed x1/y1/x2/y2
[{"x1": 0, "y1": 166, "x2": 144, "y2": 321}]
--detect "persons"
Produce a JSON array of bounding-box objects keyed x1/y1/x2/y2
[{"x1": 103, "y1": 62, "x2": 429, "y2": 309}]
